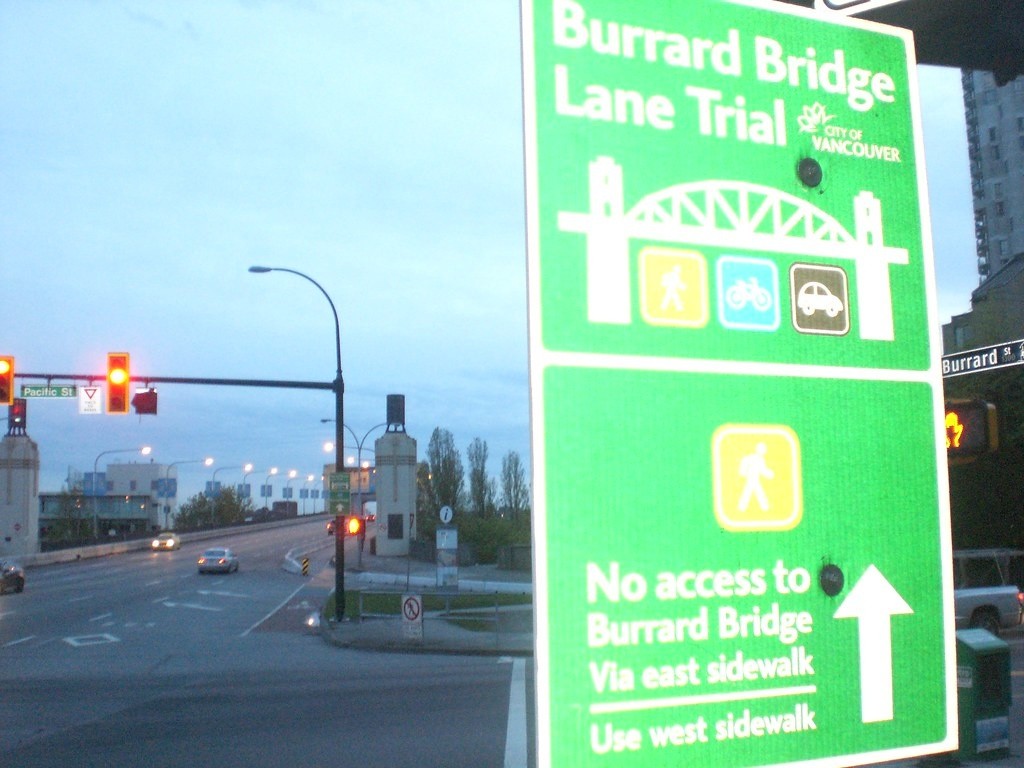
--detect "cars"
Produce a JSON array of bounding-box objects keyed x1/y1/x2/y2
[
  {"x1": 367, "y1": 515, "x2": 374, "y2": 522},
  {"x1": 197, "y1": 547, "x2": 239, "y2": 575},
  {"x1": 328, "y1": 520, "x2": 335, "y2": 535},
  {"x1": 0, "y1": 560, "x2": 24, "y2": 595},
  {"x1": 152, "y1": 533, "x2": 180, "y2": 551}
]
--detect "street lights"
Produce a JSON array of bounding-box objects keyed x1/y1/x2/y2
[
  {"x1": 243, "y1": 467, "x2": 277, "y2": 521},
  {"x1": 93, "y1": 447, "x2": 151, "y2": 539},
  {"x1": 265, "y1": 470, "x2": 295, "y2": 519},
  {"x1": 303, "y1": 476, "x2": 325, "y2": 516},
  {"x1": 212, "y1": 463, "x2": 253, "y2": 530},
  {"x1": 287, "y1": 475, "x2": 313, "y2": 518},
  {"x1": 250, "y1": 266, "x2": 345, "y2": 622},
  {"x1": 166, "y1": 458, "x2": 213, "y2": 531},
  {"x1": 321, "y1": 419, "x2": 403, "y2": 563}
]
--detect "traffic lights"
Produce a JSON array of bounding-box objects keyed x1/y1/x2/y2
[
  {"x1": 106, "y1": 353, "x2": 130, "y2": 416},
  {"x1": 8, "y1": 399, "x2": 26, "y2": 428},
  {"x1": 0, "y1": 356, "x2": 13, "y2": 405}
]
